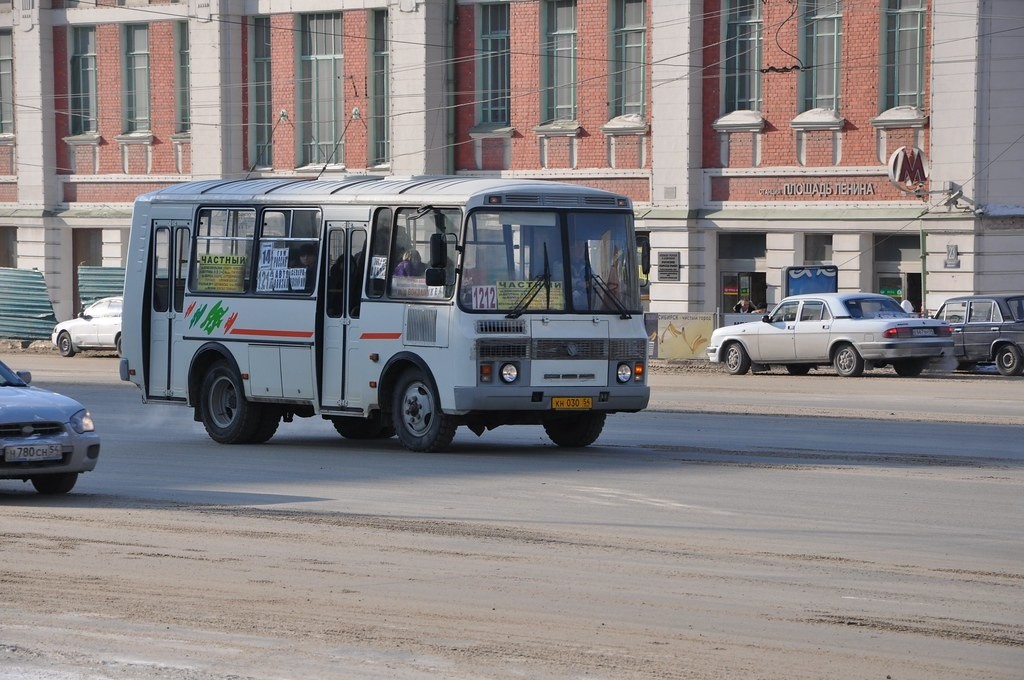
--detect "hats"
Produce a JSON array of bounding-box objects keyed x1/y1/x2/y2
[{"x1": 295, "y1": 244, "x2": 318, "y2": 257}]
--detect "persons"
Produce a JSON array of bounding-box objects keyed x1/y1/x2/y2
[
  {"x1": 395, "y1": 249, "x2": 422, "y2": 278},
  {"x1": 732, "y1": 299, "x2": 767, "y2": 313},
  {"x1": 331, "y1": 253, "x2": 362, "y2": 312},
  {"x1": 552, "y1": 238, "x2": 592, "y2": 301},
  {"x1": 289, "y1": 243, "x2": 318, "y2": 293}
]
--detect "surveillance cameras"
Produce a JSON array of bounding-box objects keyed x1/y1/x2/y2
[{"x1": 946, "y1": 191, "x2": 963, "y2": 207}]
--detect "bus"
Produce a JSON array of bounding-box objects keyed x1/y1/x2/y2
[{"x1": 119, "y1": 174, "x2": 650, "y2": 454}]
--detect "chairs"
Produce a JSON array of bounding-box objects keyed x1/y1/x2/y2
[
  {"x1": 800, "y1": 307, "x2": 812, "y2": 320},
  {"x1": 849, "y1": 306, "x2": 862, "y2": 317}
]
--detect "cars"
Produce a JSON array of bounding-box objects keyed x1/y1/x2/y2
[
  {"x1": 930, "y1": 292, "x2": 1024, "y2": 379},
  {"x1": 51, "y1": 296, "x2": 124, "y2": 358},
  {"x1": 0, "y1": 361, "x2": 100, "y2": 495},
  {"x1": 706, "y1": 292, "x2": 955, "y2": 378}
]
